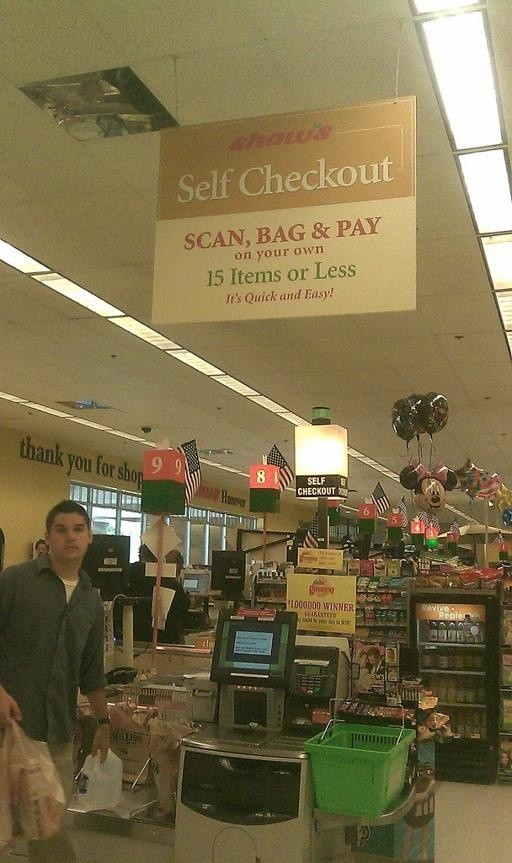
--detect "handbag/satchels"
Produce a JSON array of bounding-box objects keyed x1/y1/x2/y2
[{"x1": 74, "y1": 701, "x2": 195, "y2": 819}]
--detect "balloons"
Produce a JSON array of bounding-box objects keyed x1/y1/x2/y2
[
  {"x1": 400, "y1": 458, "x2": 457, "y2": 515},
  {"x1": 392, "y1": 392, "x2": 449, "y2": 448},
  {"x1": 455, "y1": 456, "x2": 512, "y2": 528}
]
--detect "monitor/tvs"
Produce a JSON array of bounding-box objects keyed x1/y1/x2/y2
[
  {"x1": 82, "y1": 534, "x2": 131, "y2": 584},
  {"x1": 210, "y1": 609, "x2": 298, "y2": 690},
  {"x1": 210, "y1": 549, "x2": 245, "y2": 591},
  {"x1": 180, "y1": 569, "x2": 211, "y2": 596}
]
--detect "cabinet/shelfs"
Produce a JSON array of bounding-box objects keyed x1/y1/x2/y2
[{"x1": 252, "y1": 569, "x2": 512, "y2": 786}]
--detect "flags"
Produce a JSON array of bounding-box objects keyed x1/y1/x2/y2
[
  {"x1": 493, "y1": 531, "x2": 505, "y2": 543},
  {"x1": 174, "y1": 440, "x2": 202, "y2": 507},
  {"x1": 371, "y1": 481, "x2": 390, "y2": 516},
  {"x1": 428, "y1": 514, "x2": 441, "y2": 533},
  {"x1": 398, "y1": 497, "x2": 409, "y2": 527},
  {"x1": 303, "y1": 529, "x2": 319, "y2": 549},
  {"x1": 449, "y1": 520, "x2": 460, "y2": 534},
  {"x1": 414, "y1": 508, "x2": 430, "y2": 526},
  {"x1": 266, "y1": 444, "x2": 295, "y2": 493}
]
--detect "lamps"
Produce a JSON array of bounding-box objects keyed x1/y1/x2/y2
[
  {"x1": 141, "y1": 450, "x2": 186, "y2": 665},
  {"x1": 248, "y1": 424, "x2": 509, "y2": 561}
]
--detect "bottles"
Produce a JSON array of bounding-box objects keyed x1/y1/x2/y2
[
  {"x1": 429, "y1": 619, "x2": 485, "y2": 643},
  {"x1": 427, "y1": 675, "x2": 486, "y2": 703},
  {"x1": 438, "y1": 707, "x2": 492, "y2": 740},
  {"x1": 463, "y1": 614, "x2": 473, "y2": 637},
  {"x1": 421, "y1": 649, "x2": 484, "y2": 671}
]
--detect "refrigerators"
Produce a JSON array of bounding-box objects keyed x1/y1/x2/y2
[{"x1": 409, "y1": 590, "x2": 498, "y2": 785}]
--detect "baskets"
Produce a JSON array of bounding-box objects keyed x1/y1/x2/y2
[{"x1": 305, "y1": 722, "x2": 417, "y2": 820}]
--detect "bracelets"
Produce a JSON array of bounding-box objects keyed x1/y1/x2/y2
[{"x1": 96, "y1": 716, "x2": 110, "y2": 725}]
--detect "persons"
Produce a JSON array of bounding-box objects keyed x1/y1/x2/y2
[
  {"x1": 125, "y1": 544, "x2": 158, "y2": 641},
  {"x1": 35, "y1": 540, "x2": 50, "y2": 559},
  {"x1": 157, "y1": 550, "x2": 190, "y2": 644},
  {"x1": 356, "y1": 648, "x2": 395, "y2": 682},
  {"x1": 0, "y1": 501, "x2": 112, "y2": 863}
]
